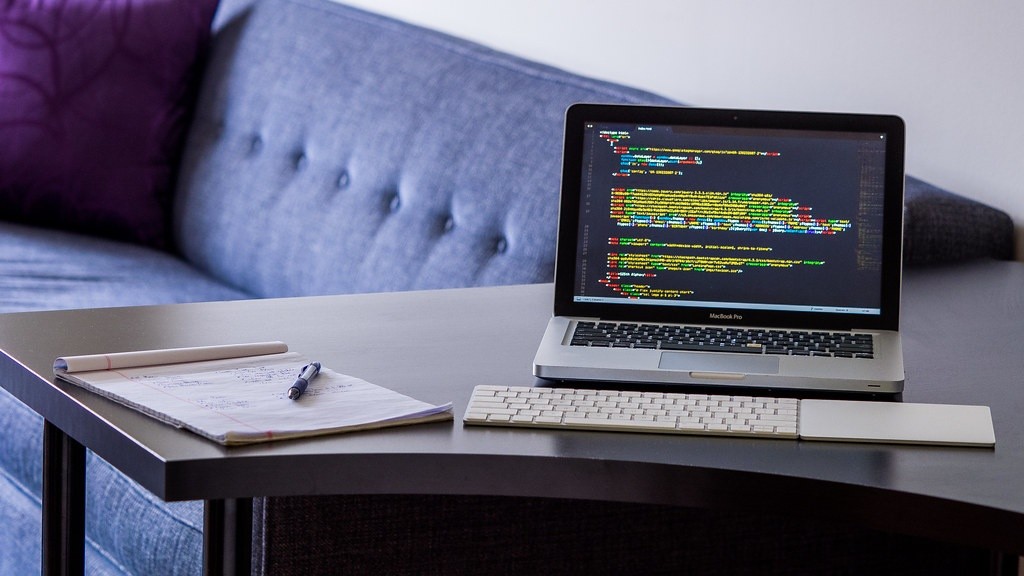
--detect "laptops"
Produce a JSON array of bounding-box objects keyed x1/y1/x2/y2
[{"x1": 532, "y1": 102, "x2": 904, "y2": 393}]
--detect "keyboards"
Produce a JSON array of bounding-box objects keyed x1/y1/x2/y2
[{"x1": 463, "y1": 384, "x2": 995, "y2": 449}]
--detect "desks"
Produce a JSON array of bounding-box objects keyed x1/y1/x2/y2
[{"x1": 2, "y1": 254, "x2": 1022, "y2": 572}]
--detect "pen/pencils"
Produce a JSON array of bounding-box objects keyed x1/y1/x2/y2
[{"x1": 286, "y1": 360, "x2": 322, "y2": 401}]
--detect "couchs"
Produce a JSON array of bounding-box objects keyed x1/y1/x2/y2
[{"x1": 1, "y1": 0, "x2": 1021, "y2": 574}]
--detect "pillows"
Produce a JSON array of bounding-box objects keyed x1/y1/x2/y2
[{"x1": 0, "y1": 1, "x2": 217, "y2": 254}]
